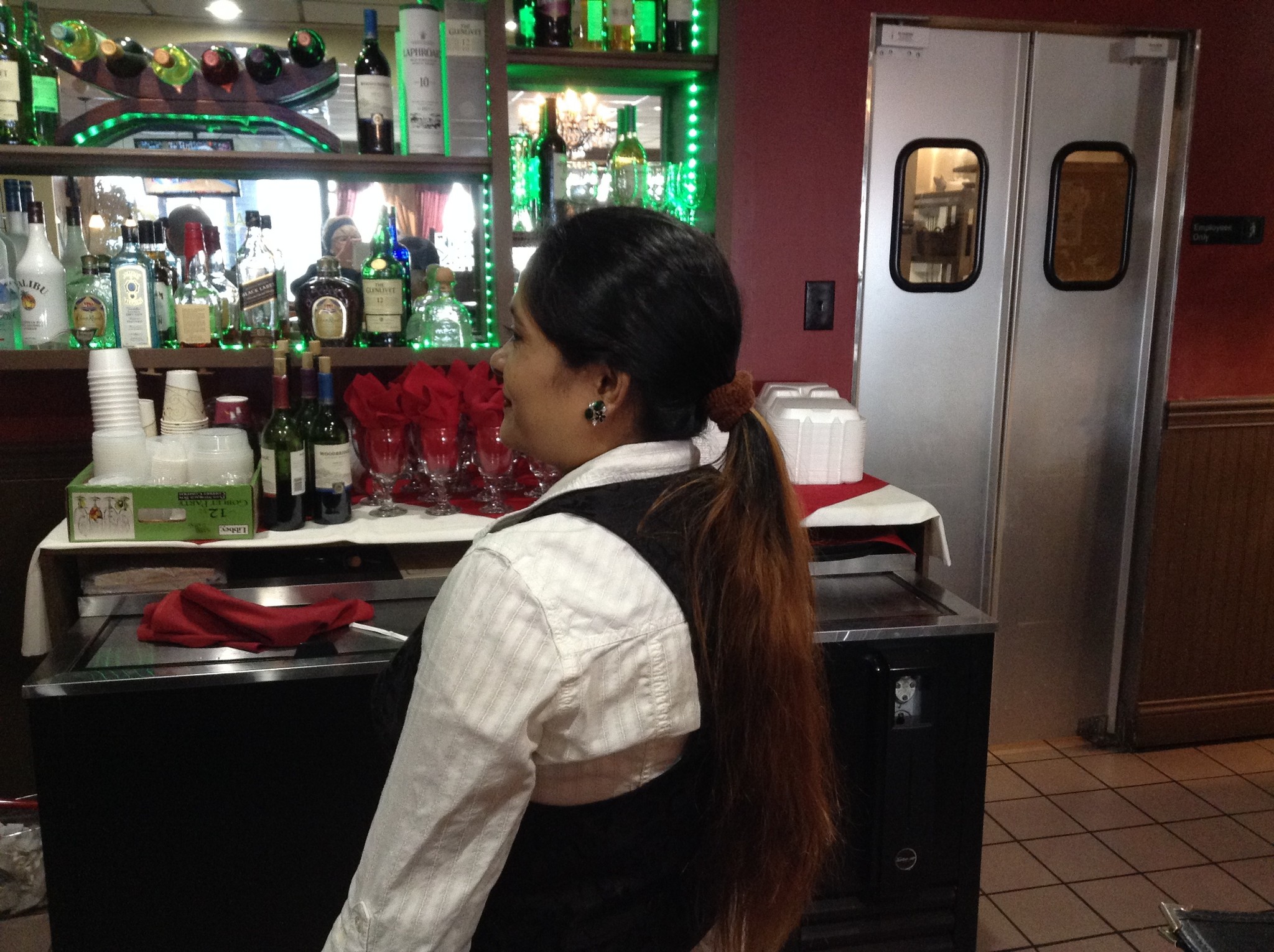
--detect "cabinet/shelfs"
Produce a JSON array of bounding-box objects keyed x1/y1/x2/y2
[{"x1": 0, "y1": 1, "x2": 719, "y2": 443}]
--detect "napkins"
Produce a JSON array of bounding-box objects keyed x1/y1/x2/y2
[{"x1": 341, "y1": 363, "x2": 545, "y2": 490}]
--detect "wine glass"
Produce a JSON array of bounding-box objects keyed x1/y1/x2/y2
[
  {"x1": 510, "y1": 136, "x2": 709, "y2": 231},
  {"x1": 362, "y1": 420, "x2": 561, "y2": 521}
]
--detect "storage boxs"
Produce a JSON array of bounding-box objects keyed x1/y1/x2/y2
[{"x1": 63, "y1": 459, "x2": 262, "y2": 543}]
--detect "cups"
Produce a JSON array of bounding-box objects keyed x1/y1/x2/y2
[{"x1": 86, "y1": 347, "x2": 258, "y2": 493}]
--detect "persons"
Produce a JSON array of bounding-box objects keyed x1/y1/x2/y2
[
  {"x1": 166, "y1": 205, "x2": 440, "y2": 302},
  {"x1": 325, "y1": 204, "x2": 828, "y2": 952}
]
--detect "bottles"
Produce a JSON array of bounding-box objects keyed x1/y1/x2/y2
[
  {"x1": 530, "y1": 96, "x2": 648, "y2": 228},
  {"x1": 506, "y1": 0, "x2": 700, "y2": 57},
  {"x1": 0, "y1": 2, "x2": 35, "y2": 143},
  {"x1": 20, "y1": 1, "x2": 61, "y2": 147},
  {"x1": 352, "y1": 9, "x2": 396, "y2": 154},
  {"x1": 244, "y1": 43, "x2": 285, "y2": 82},
  {"x1": 2, "y1": 175, "x2": 476, "y2": 357},
  {"x1": 197, "y1": 45, "x2": 242, "y2": 84},
  {"x1": 49, "y1": 16, "x2": 109, "y2": 63},
  {"x1": 259, "y1": 337, "x2": 353, "y2": 531},
  {"x1": 101, "y1": 34, "x2": 155, "y2": 77},
  {"x1": 285, "y1": 28, "x2": 325, "y2": 67},
  {"x1": 150, "y1": 44, "x2": 199, "y2": 86}
]
